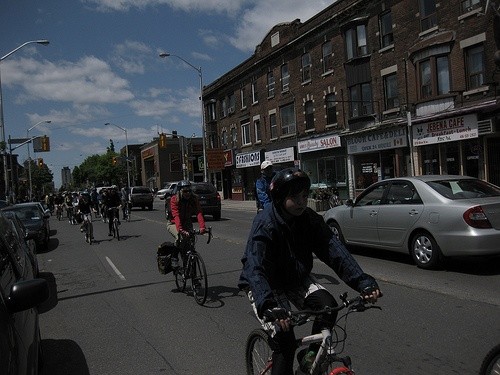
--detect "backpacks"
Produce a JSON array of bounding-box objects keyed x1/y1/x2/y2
[{"x1": 157, "y1": 242, "x2": 177, "y2": 274}]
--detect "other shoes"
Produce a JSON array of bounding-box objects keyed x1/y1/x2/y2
[
  {"x1": 171, "y1": 260, "x2": 179, "y2": 269},
  {"x1": 297, "y1": 349, "x2": 316, "y2": 375},
  {"x1": 91, "y1": 238, "x2": 94, "y2": 241},
  {"x1": 81, "y1": 228, "x2": 83, "y2": 233},
  {"x1": 195, "y1": 282, "x2": 201, "y2": 287}
]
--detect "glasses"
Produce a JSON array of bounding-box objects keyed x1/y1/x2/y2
[
  {"x1": 289, "y1": 194, "x2": 309, "y2": 200},
  {"x1": 180, "y1": 189, "x2": 192, "y2": 193}
]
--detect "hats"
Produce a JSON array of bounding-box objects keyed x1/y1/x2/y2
[{"x1": 261, "y1": 160, "x2": 273, "y2": 169}]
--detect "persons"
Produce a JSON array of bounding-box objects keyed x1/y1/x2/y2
[
  {"x1": 256, "y1": 161, "x2": 276, "y2": 208},
  {"x1": 238, "y1": 169, "x2": 380, "y2": 375},
  {"x1": 166, "y1": 180, "x2": 207, "y2": 289},
  {"x1": 45, "y1": 183, "x2": 131, "y2": 240}
]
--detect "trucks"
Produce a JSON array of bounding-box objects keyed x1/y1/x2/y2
[{"x1": 96, "y1": 185, "x2": 117, "y2": 194}]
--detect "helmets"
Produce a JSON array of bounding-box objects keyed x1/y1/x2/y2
[
  {"x1": 110, "y1": 184, "x2": 117, "y2": 189},
  {"x1": 269, "y1": 167, "x2": 311, "y2": 191},
  {"x1": 177, "y1": 180, "x2": 191, "y2": 190},
  {"x1": 83, "y1": 191, "x2": 89, "y2": 196}
]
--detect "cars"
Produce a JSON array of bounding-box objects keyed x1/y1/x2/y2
[
  {"x1": 62, "y1": 191, "x2": 83, "y2": 196},
  {"x1": 0, "y1": 211, "x2": 49, "y2": 375},
  {"x1": 9, "y1": 204, "x2": 50, "y2": 247},
  {"x1": 322, "y1": 174, "x2": 500, "y2": 270},
  {"x1": 18, "y1": 202, "x2": 50, "y2": 214}
]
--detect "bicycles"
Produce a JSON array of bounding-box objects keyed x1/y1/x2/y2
[
  {"x1": 50, "y1": 205, "x2": 74, "y2": 225},
  {"x1": 478, "y1": 343, "x2": 500, "y2": 375},
  {"x1": 107, "y1": 206, "x2": 123, "y2": 239},
  {"x1": 125, "y1": 201, "x2": 130, "y2": 222},
  {"x1": 79, "y1": 211, "x2": 97, "y2": 245},
  {"x1": 174, "y1": 226, "x2": 213, "y2": 305},
  {"x1": 245, "y1": 292, "x2": 384, "y2": 374},
  {"x1": 328, "y1": 183, "x2": 343, "y2": 209},
  {"x1": 101, "y1": 203, "x2": 107, "y2": 223}
]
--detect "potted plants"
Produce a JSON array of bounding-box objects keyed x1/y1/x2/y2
[{"x1": 308, "y1": 189, "x2": 331, "y2": 211}]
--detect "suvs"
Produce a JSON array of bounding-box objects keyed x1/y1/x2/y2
[
  {"x1": 165, "y1": 181, "x2": 221, "y2": 219},
  {"x1": 129, "y1": 186, "x2": 153, "y2": 209},
  {"x1": 157, "y1": 181, "x2": 194, "y2": 200}
]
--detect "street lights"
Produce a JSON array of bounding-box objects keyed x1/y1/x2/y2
[
  {"x1": 8, "y1": 133, "x2": 47, "y2": 204},
  {"x1": 159, "y1": 53, "x2": 207, "y2": 183},
  {"x1": 0, "y1": 39, "x2": 52, "y2": 202},
  {"x1": 27, "y1": 120, "x2": 52, "y2": 203},
  {"x1": 104, "y1": 122, "x2": 131, "y2": 189}
]
